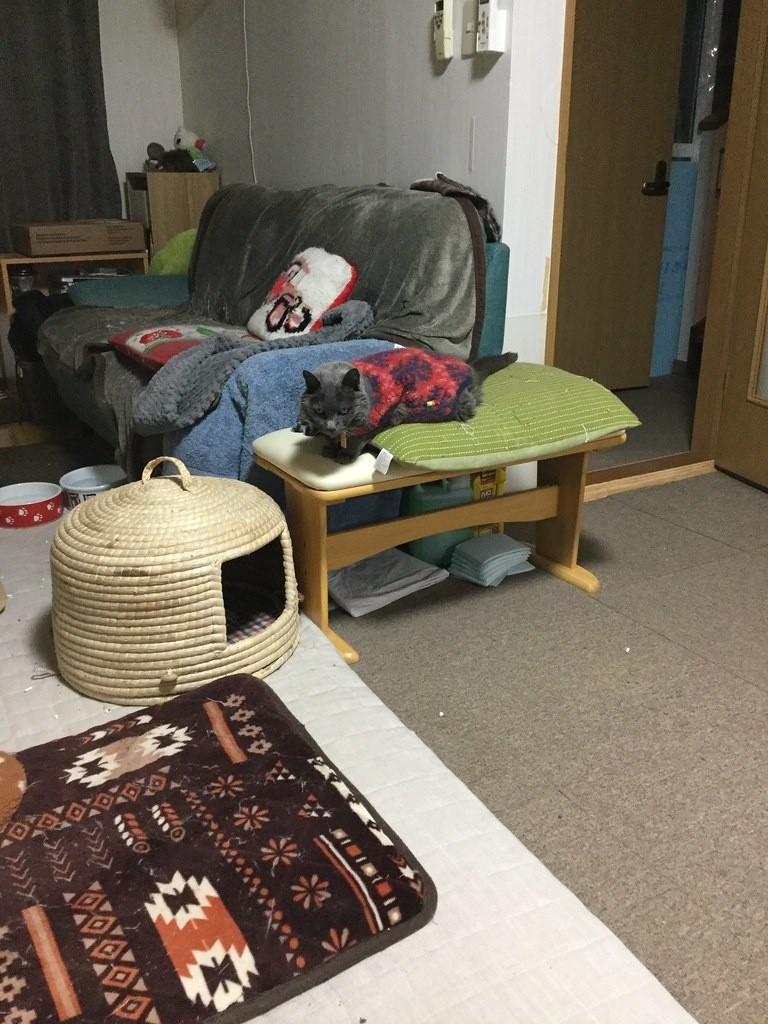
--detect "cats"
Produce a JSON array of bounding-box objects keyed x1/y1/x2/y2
[{"x1": 291, "y1": 348, "x2": 519, "y2": 464}]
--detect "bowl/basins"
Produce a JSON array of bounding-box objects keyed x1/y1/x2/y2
[
  {"x1": 0, "y1": 481, "x2": 64, "y2": 530},
  {"x1": 58, "y1": 464, "x2": 132, "y2": 512}
]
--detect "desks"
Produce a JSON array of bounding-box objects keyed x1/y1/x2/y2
[
  {"x1": 0, "y1": 508, "x2": 698, "y2": 1024},
  {"x1": 251, "y1": 433, "x2": 628, "y2": 665}
]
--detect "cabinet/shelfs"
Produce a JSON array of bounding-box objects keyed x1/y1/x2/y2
[
  {"x1": 1, "y1": 249, "x2": 149, "y2": 319},
  {"x1": 125, "y1": 171, "x2": 222, "y2": 264}
]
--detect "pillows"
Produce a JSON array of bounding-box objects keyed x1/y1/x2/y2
[
  {"x1": 107, "y1": 324, "x2": 252, "y2": 372},
  {"x1": 245, "y1": 246, "x2": 358, "y2": 342},
  {"x1": 1, "y1": 673, "x2": 438, "y2": 1024},
  {"x1": 252, "y1": 426, "x2": 377, "y2": 492},
  {"x1": 369, "y1": 360, "x2": 644, "y2": 475}
]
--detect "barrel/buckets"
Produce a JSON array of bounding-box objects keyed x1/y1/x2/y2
[{"x1": 402, "y1": 474, "x2": 474, "y2": 568}]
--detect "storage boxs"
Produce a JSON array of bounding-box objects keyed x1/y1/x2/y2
[
  {"x1": 0, "y1": 481, "x2": 64, "y2": 528},
  {"x1": 59, "y1": 463, "x2": 128, "y2": 510},
  {"x1": 9, "y1": 218, "x2": 147, "y2": 257},
  {"x1": 50, "y1": 456, "x2": 299, "y2": 708}
]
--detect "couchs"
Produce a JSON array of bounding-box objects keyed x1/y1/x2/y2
[{"x1": 36, "y1": 175, "x2": 512, "y2": 591}]
